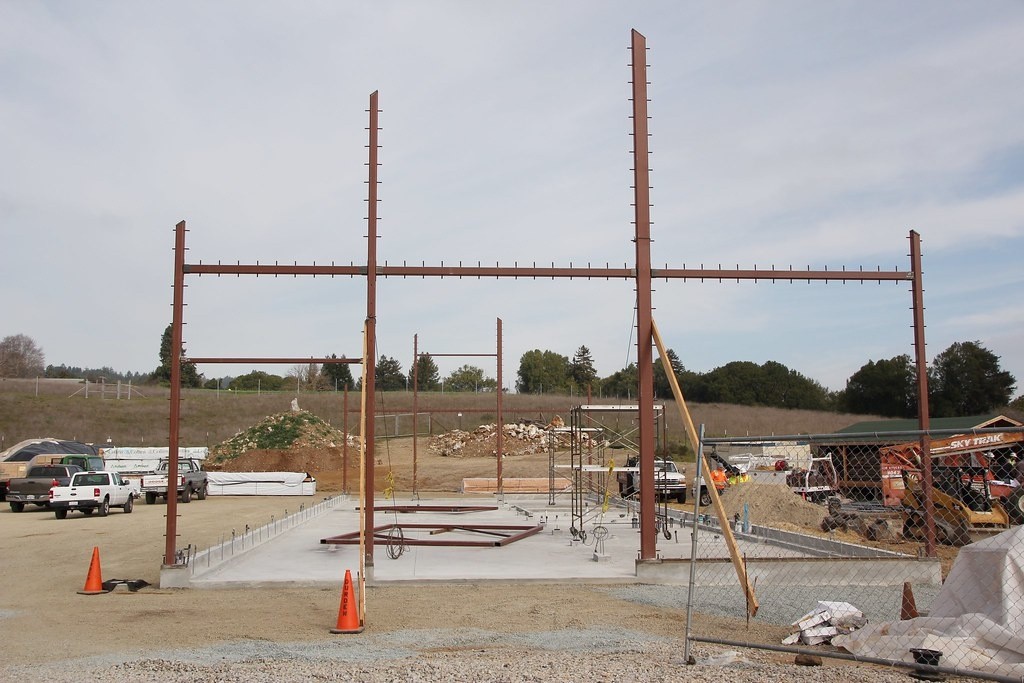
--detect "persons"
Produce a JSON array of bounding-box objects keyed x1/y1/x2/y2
[
  {"x1": 986, "y1": 450, "x2": 1024, "y2": 485},
  {"x1": 711, "y1": 463, "x2": 752, "y2": 496}
]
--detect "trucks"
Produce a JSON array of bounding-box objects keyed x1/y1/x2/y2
[
  {"x1": 0, "y1": 453, "x2": 105, "y2": 499},
  {"x1": 691, "y1": 452, "x2": 839, "y2": 506}
]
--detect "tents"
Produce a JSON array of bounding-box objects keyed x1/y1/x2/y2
[{"x1": 0, "y1": 438, "x2": 117, "y2": 462}]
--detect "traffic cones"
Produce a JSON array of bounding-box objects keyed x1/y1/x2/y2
[
  {"x1": 329, "y1": 570, "x2": 365, "y2": 634},
  {"x1": 77, "y1": 547, "x2": 108, "y2": 596},
  {"x1": 900, "y1": 582, "x2": 919, "y2": 621}
]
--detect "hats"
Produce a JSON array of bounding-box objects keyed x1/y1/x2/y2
[{"x1": 717, "y1": 463, "x2": 723, "y2": 468}]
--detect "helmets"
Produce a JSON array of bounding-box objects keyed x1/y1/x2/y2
[
  {"x1": 1010, "y1": 452, "x2": 1017, "y2": 457},
  {"x1": 985, "y1": 452, "x2": 995, "y2": 458},
  {"x1": 741, "y1": 468, "x2": 747, "y2": 473}
]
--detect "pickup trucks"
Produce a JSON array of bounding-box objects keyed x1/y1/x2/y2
[
  {"x1": 140, "y1": 457, "x2": 209, "y2": 504},
  {"x1": 49, "y1": 470, "x2": 134, "y2": 519},
  {"x1": 6, "y1": 464, "x2": 95, "y2": 513},
  {"x1": 617, "y1": 459, "x2": 687, "y2": 504}
]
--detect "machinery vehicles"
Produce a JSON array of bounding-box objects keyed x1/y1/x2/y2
[
  {"x1": 899, "y1": 465, "x2": 1009, "y2": 545},
  {"x1": 880, "y1": 431, "x2": 1024, "y2": 523}
]
